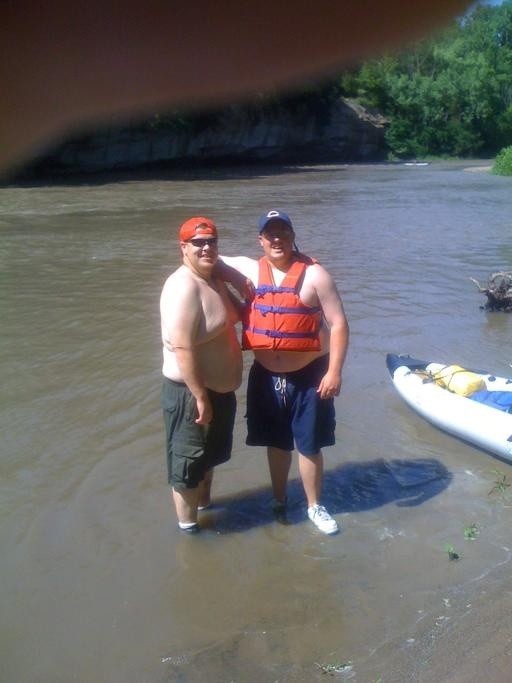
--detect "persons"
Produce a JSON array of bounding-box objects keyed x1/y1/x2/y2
[
  {"x1": 212, "y1": 211, "x2": 351, "y2": 535},
  {"x1": 158, "y1": 217, "x2": 246, "y2": 535}
]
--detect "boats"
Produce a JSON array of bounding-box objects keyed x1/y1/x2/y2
[{"x1": 386, "y1": 353, "x2": 512, "y2": 463}]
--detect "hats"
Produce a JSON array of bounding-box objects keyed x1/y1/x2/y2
[
  {"x1": 179, "y1": 218, "x2": 218, "y2": 242},
  {"x1": 258, "y1": 210, "x2": 294, "y2": 236}
]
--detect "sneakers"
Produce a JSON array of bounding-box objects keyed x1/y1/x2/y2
[{"x1": 307, "y1": 504, "x2": 338, "y2": 533}]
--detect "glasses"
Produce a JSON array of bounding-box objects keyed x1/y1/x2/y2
[
  {"x1": 263, "y1": 231, "x2": 290, "y2": 239},
  {"x1": 186, "y1": 238, "x2": 217, "y2": 246}
]
